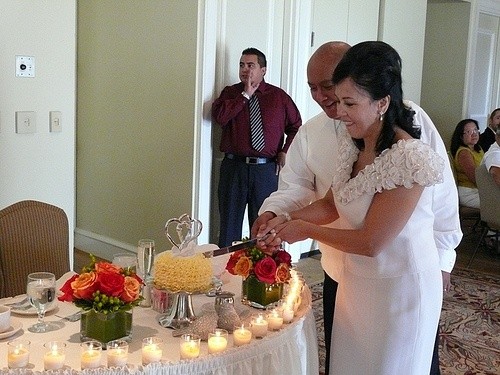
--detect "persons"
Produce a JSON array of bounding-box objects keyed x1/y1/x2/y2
[
  {"x1": 250, "y1": 41, "x2": 463, "y2": 375},
  {"x1": 258, "y1": 41, "x2": 444, "y2": 375},
  {"x1": 211, "y1": 47, "x2": 303, "y2": 248},
  {"x1": 451, "y1": 118, "x2": 484, "y2": 210},
  {"x1": 474, "y1": 107, "x2": 500, "y2": 153},
  {"x1": 480, "y1": 130, "x2": 500, "y2": 186}
]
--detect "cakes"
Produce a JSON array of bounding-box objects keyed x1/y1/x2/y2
[{"x1": 152, "y1": 249, "x2": 213, "y2": 293}]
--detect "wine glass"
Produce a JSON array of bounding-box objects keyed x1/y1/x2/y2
[
  {"x1": 137, "y1": 239, "x2": 155, "y2": 281},
  {"x1": 27, "y1": 272, "x2": 56, "y2": 333}
]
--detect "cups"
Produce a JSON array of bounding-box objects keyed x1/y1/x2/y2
[
  {"x1": 112, "y1": 253, "x2": 137, "y2": 272},
  {"x1": 233, "y1": 321, "x2": 252, "y2": 348},
  {"x1": 180, "y1": 334, "x2": 201, "y2": 360},
  {"x1": 43, "y1": 341, "x2": 66, "y2": 371},
  {"x1": 0, "y1": 305, "x2": 11, "y2": 333},
  {"x1": 208, "y1": 329, "x2": 229, "y2": 355},
  {"x1": 142, "y1": 337, "x2": 163, "y2": 365},
  {"x1": 206, "y1": 278, "x2": 222, "y2": 297},
  {"x1": 250, "y1": 312, "x2": 269, "y2": 339},
  {"x1": 107, "y1": 340, "x2": 128, "y2": 368},
  {"x1": 80, "y1": 340, "x2": 102, "y2": 370},
  {"x1": 7, "y1": 339, "x2": 31, "y2": 369},
  {"x1": 266, "y1": 263, "x2": 306, "y2": 332},
  {"x1": 232, "y1": 241, "x2": 243, "y2": 246}
]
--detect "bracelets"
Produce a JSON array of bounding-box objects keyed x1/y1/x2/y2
[
  {"x1": 282, "y1": 210, "x2": 292, "y2": 223},
  {"x1": 241, "y1": 91, "x2": 250, "y2": 99}
]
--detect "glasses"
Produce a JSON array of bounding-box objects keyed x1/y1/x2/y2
[{"x1": 463, "y1": 129, "x2": 480, "y2": 136}]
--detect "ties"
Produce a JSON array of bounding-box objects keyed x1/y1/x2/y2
[{"x1": 249, "y1": 95, "x2": 266, "y2": 153}]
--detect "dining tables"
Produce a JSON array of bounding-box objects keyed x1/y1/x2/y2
[{"x1": 0, "y1": 254, "x2": 318, "y2": 375}]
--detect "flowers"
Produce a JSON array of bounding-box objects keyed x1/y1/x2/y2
[
  {"x1": 59, "y1": 251, "x2": 145, "y2": 313},
  {"x1": 227, "y1": 243, "x2": 294, "y2": 287}
]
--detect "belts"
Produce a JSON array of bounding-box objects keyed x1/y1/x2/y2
[{"x1": 225, "y1": 153, "x2": 273, "y2": 164}]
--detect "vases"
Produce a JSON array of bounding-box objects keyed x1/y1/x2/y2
[
  {"x1": 240, "y1": 278, "x2": 286, "y2": 310},
  {"x1": 80, "y1": 309, "x2": 132, "y2": 349}
]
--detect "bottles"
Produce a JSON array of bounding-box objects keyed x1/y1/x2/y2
[{"x1": 215, "y1": 292, "x2": 234, "y2": 312}]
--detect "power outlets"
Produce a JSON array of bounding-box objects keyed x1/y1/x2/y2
[
  {"x1": 50, "y1": 111, "x2": 62, "y2": 133},
  {"x1": 15, "y1": 111, "x2": 38, "y2": 135}
]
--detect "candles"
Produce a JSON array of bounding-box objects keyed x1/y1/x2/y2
[
  {"x1": 81, "y1": 346, "x2": 101, "y2": 371},
  {"x1": 8, "y1": 346, "x2": 29, "y2": 368},
  {"x1": 143, "y1": 344, "x2": 161, "y2": 365},
  {"x1": 180, "y1": 342, "x2": 199, "y2": 360},
  {"x1": 45, "y1": 349, "x2": 64, "y2": 370},
  {"x1": 207, "y1": 335, "x2": 226, "y2": 354},
  {"x1": 108, "y1": 347, "x2": 128, "y2": 367},
  {"x1": 234, "y1": 272, "x2": 307, "y2": 347}
]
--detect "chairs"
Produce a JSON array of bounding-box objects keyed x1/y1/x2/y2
[
  {"x1": 0, "y1": 200, "x2": 71, "y2": 299},
  {"x1": 447, "y1": 151, "x2": 500, "y2": 268}
]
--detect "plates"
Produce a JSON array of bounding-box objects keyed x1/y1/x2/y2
[
  {"x1": 4, "y1": 297, "x2": 57, "y2": 314},
  {"x1": 0, "y1": 317, "x2": 23, "y2": 339}
]
